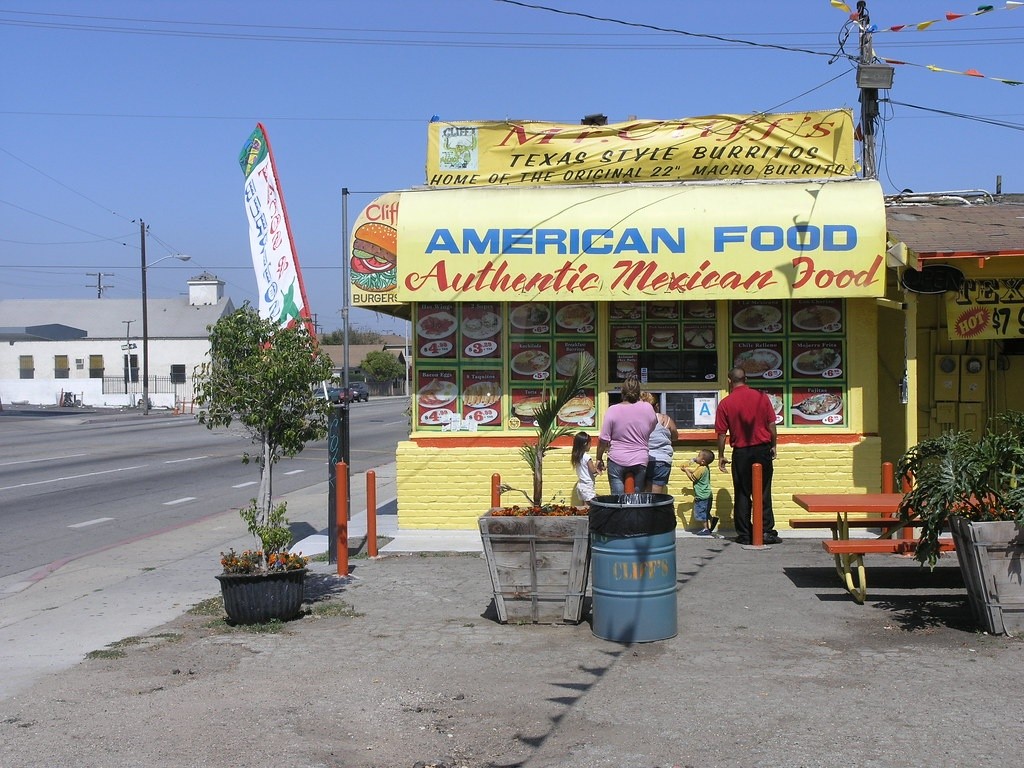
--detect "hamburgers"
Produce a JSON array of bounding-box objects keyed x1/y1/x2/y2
[
  {"x1": 689, "y1": 304, "x2": 713, "y2": 318},
  {"x1": 617, "y1": 361, "x2": 636, "y2": 379},
  {"x1": 609, "y1": 302, "x2": 676, "y2": 348}
]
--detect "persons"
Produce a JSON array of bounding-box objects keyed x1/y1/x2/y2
[
  {"x1": 715, "y1": 368, "x2": 782, "y2": 544},
  {"x1": 639, "y1": 390, "x2": 679, "y2": 492},
  {"x1": 571, "y1": 431, "x2": 598, "y2": 506},
  {"x1": 596, "y1": 376, "x2": 658, "y2": 495},
  {"x1": 680, "y1": 449, "x2": 719, "y2": 535}
]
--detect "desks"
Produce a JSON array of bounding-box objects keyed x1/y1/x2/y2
[{"x1": 792, "y1": 492, "x2": 1018, "y2": 593}]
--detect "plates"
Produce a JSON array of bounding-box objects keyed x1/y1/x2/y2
[
  {"x1": 792, "y1": 306, "x2": 840, "y2": 330},
  {"x1": 734, "y1": 305, "x2": 782, "y2": 330},
  {"x1": 417, "y1": 312, "x2": 457, "y2": 340},
  {"x1": 462, "y1": 382, "x2": 501, "y2": 408},
  {"x1": 555, "y1": 304, "x2": 593, "y2": 329},
  {"x1": 462, "y1": 312, "x2": 503, "y2": 340},
  {"x1": 734, "y1": 349, "x2": 782, "y2": 376},
  {"x1": 559, "y1": 407, "x2": 595, "y2": 423},
  {"x1": 556, "y1": 353, "x2": 595, "y2": 376},
  {"x1": 510, "y1": 302, "x2": 550, "y2": 329},
  {"x1": 417, "y1": 381, "x2": 457, "y2": 408},
  {"x1": 792, "y1": 349, "x2": 841, "y2": 375},
  {"x1": 510, "y1": 350, "x2": 551, "y2": 375},
  {"x1": 767, "y1": 394, "x2": 783, "y2": 415}
]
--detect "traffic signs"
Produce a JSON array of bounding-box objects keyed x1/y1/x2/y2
[{"x1": 121, "y1": 343, "x2": 137, "y2": 350}]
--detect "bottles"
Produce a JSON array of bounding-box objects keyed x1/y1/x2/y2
[{"x1": 681, "y1": 457, "x2": 697, "y2": 470}]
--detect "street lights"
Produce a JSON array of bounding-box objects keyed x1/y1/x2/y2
[{"x1": 142, "y1": 254, "x2": 191, "y2": 416}]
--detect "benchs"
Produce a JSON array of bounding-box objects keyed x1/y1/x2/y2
[{"x1": 789, "y1": 519, "x2": 957, "y2": 601}]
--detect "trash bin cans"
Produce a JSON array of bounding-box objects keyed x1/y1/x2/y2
[{"x1": 585, "y1": 491, "x2": 678, "y2": 644}]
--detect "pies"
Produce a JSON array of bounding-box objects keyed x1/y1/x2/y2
[{"x1": 684, "y1": 329, "x2": 713, "y2": 347}]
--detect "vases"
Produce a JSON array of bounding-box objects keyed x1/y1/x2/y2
[
  {"x1": 215, "y1": 567, "x2": 308, "y2": 626},
  {"x1": 948, "y1": 515, "x2": 1024, "y2": 639},
  {"x1": 477, "y1": 505, "x2": 591, "y2": 626}
]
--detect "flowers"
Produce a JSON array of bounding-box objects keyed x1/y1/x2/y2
[
  {"x1": 895, "y1": 409, "x2": 1024, "y2": 573},
  {"x1": 190, "y1": 299, "x2": 340, "y2": 574},
  {"x1": 477, "y1": 351, "x2": 599, "y2": 517}
]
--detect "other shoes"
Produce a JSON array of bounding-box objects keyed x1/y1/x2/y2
[
  {"x1": 710, "y1": 517, "x2": 719, "y2": 531},
  {"x1": 697, "y1": 528, "x2": 711, "y2": 535},
  {"x1": 763, "y1": 530, "x2": 782, "y2": 544},
  {"x1": 736, "y1": 535, "x2": 752, "y2": 545}
]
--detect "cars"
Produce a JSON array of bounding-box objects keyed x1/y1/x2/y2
[{"x1": 311, "y1": 381, "x2": 370, "y2": 405}]
